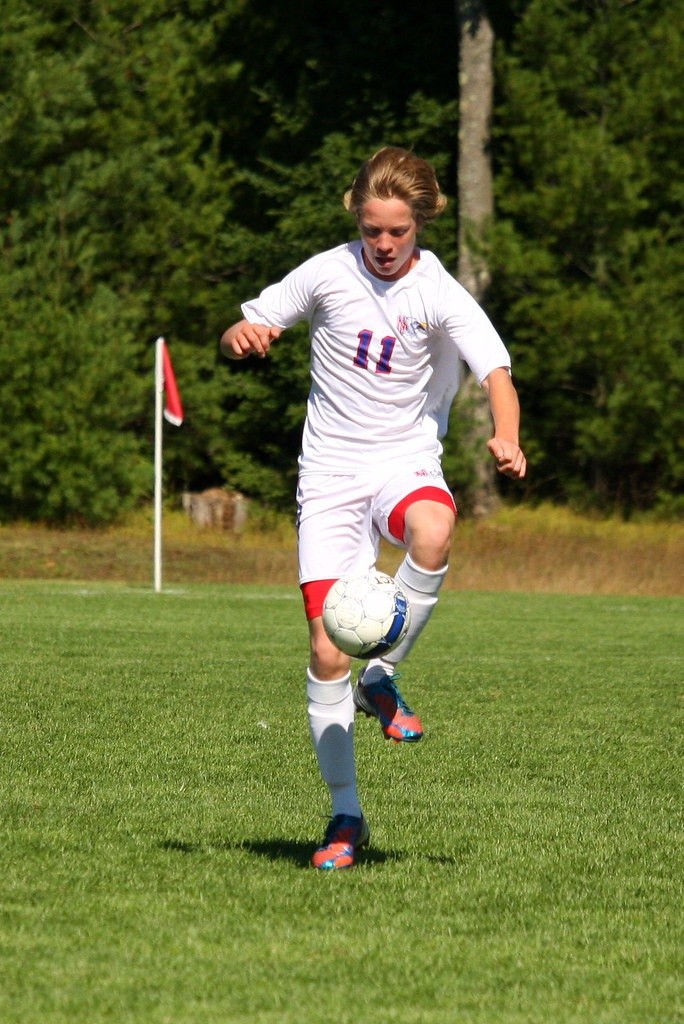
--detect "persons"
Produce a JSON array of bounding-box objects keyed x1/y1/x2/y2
[{"x1": 218, "y1": 146, "x2": 529, "y2": 869}]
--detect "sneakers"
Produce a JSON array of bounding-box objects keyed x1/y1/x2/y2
[
  {"x1": 352, "y1": 667, "x2": 424, "y2": 744},
  {"x1": 309, "y1": 811, "x2": 371, "y2": 872}
]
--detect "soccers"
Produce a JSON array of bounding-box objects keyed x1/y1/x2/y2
[{"x1": 322, "y1": 570, "x2": 410, "y2": 660}]
